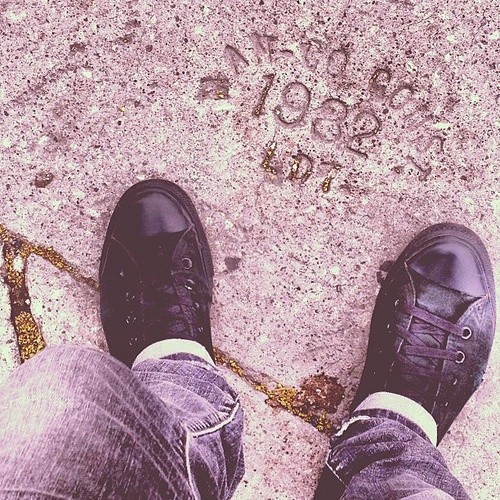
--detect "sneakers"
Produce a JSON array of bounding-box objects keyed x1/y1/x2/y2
[
  {"x1": 96, "y1": 179, "x2": 220, "y2": 373},
  {"x1": 349, "y1": 222, "x2": 497, "y2": 449}
]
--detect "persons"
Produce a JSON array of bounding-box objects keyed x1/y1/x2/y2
[{"x1": 0, "y1": 180, "x2": 496, "y2": 500}]
